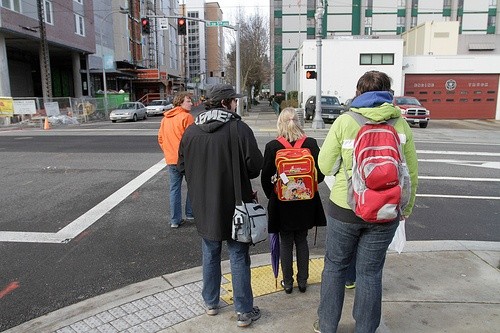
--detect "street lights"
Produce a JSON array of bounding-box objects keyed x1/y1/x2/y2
[
  {"x1": 312, "y1": 4, "x2": 325, "y2": 129},
  {"x1": 99, "y1": 9, "x2": 130, "y2": 121}
]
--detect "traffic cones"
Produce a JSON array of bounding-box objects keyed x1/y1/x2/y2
[{"x1": 44, "y1": 117, "x2": 50, "y2": 130}]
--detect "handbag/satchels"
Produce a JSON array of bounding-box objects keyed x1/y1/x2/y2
[
  {"x1": 388, "y1": 219, "x2": 406, "y2": 255},
  {"x1": 232, "y1": 203, "x2": 268, "y2": 244}
]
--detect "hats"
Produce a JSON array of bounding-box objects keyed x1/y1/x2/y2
[{"x1": 210, "y1": 84, "x2": 246, "y2": 101}]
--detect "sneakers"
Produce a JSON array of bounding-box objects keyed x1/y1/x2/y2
[
  {"x1": 206, "y1": 304, "x2": 218, "y2": 315},
  {"x1": 236, "y1": 305, "x2": 261, "y2": 326}
]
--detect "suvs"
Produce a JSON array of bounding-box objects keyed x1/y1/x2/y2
[{"x1": 392, "y1": 96, "x2": 430, "y2": 128}]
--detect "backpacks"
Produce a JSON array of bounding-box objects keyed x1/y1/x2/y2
[
  {"x1": 273, "y1": 137, "x2": 318, "y2": 201},
  {"x1": 343, "y1": 111, "x2": 411, "y2": 224}
]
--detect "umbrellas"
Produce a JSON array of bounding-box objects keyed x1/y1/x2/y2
[{"x1": 268, "y1": 232, "x2": 279, "y2": 289}]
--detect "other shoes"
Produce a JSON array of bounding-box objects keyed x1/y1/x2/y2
[
  {"x1": 299, "y1": 286, "x2": 306, "y2": 293},
  {"x1": 281, "y1": 280, "x2": 292, "y2": 294},
  {"x1": 172, "y1": 218, "x2": 185, "y2": 228},
  {"x1": 186, "y1": 215, "x2": 195, "y2": 220},
  {"x1": 312, "y1": 318, "x2": 320, "y2": 333},
  {"x1": 345, "y1": 281, "x2": 356, "y2": 288}
]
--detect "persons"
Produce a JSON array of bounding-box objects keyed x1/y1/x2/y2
[
  {"x1": 260, "y1": 107, "x2": 328, "y2": 294},
  {"x1": 177, "y1": 85, "x2": 264, "y2": 327},
  {"x1": 253, "y1": 89, "x2": 274, "y2": 107},
  {"x1": 159, "y1": 92, "x2": 197, "y2": 227},
  {"x1": 268, "y1": 97, "x2": 272, "y2": 108},
  {"x1": 312, "y1": 71, "x2": 418, "y2": 333}
]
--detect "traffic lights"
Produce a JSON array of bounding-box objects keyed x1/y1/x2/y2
[
  {"x1": 177, "y1": 18, "x2": 187, "y2": 35},
  {"x1": 306, "y1": 71, "x2": 315, "y2": 79},
  {"x1": 141, "y1": 18, "x2": 151, "y2": 35}
]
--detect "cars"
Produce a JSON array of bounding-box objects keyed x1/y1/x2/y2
[
  {"x1": 109, "y1": 102, "x2": 147, "y2": 123},
  {"x1": 145, "y1": 100, "x2": 174, "y2": 117},
  {"x1": 305, "y1": 96, "x2": 352, "y2": 124}
]
